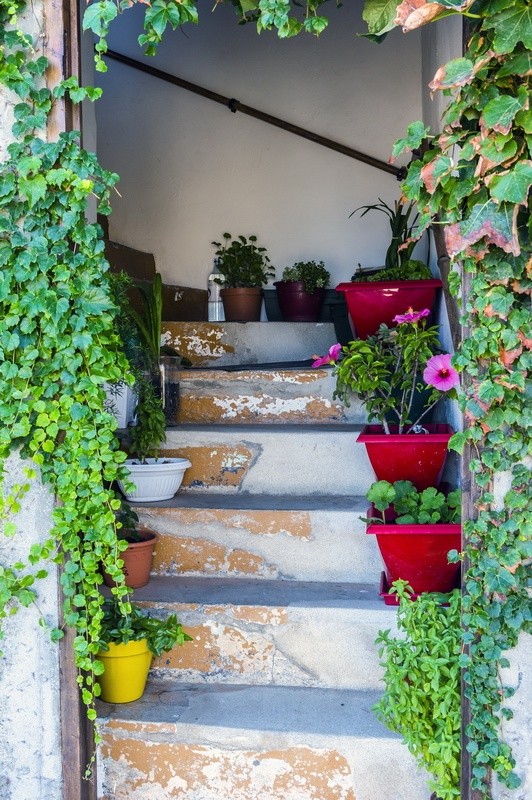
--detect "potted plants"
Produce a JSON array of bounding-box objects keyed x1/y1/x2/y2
[
  {"x1": 114, "y1": 266, "x2": 185, "y2": 430},
  {"x1": 78, "y1": 595, "x2": 196, "y2": 706},
  {"x1": 273, "y1": 258, "x2": 331, "y2": 323},
  {"x1": 115, "y1": 374, "x2": 196, "y2": 503},
  {"x1": 104, "y1": 500, "x2": 160, "y2": 591},
  {"x1": 364, "y1": 480, "x2": 464, "y2": 606},
  {"x1": 332, "y1": 195, "x2": 443, "y2": 341},
  {"x1": 210, "y1": 228, "x2": 276, "y2": 323}
]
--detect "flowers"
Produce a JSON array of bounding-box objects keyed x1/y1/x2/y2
[{"x1": 310, "y1": 304, "x2": 463, "y2": 430}]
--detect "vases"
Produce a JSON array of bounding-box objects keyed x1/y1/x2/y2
[{"x1": 353, "y1": 421, "x2": 457, "y2": 491}]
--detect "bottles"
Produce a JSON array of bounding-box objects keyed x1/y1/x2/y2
[{"x1": 207, "y1": 257, "x2": 226, "y2": 322}]
